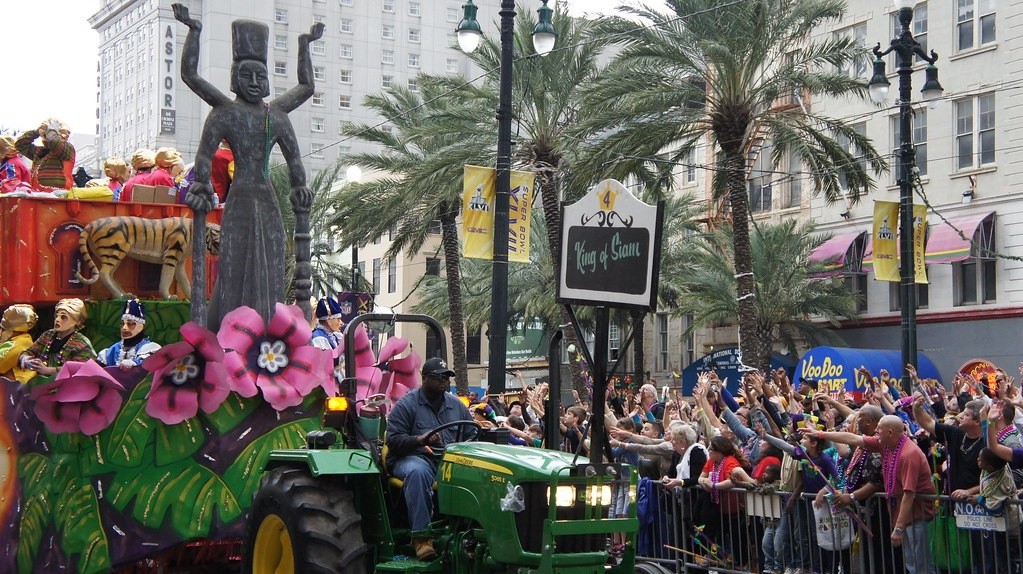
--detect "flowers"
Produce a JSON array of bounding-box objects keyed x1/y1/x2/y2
[
  {"x1": 28, "y1": 359, "x2": 126, "y2": 438},
  {"x1": 140, "y1": 319, "x2": 231, "y2": 426},
  {"x1": 378, "y1": 335, "x2": 423, "y2": 415},
  {"x1": 216, "y1": 302, "x2": 334, "y2": 412},
  {"x1": 321, "y1": 323, "x2": 383, "y2": 416}
]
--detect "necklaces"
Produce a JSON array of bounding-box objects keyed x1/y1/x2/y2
[
  {"x1": 844, "y1": 433, "x2": 868, "y2": 489},
  {"x1": 42, "y1": 337, "x2": 68, "y2": 367},
  {"x1": 979, "y1": 468, "x2": 1008, "y2": 497},
  {"x1": 711, "y1": 457, "x2": 724, "y2": 504},
  {"x1": 882, "y1": 433, "x2": 908, "y2": 505},
  {"x1": 996, "y1": 424, "x2": 1016, "y2": 443}
]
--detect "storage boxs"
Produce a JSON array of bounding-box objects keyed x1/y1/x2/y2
[
  {"x1": 154, "y1": 185, "x2": 177, "y2": 204},
  {"x1": 67, "y1": 185, "x2": 114, "y2": 202},
  {"x1": 130, "y1": 184, "x2": 155, "y2": 203}
]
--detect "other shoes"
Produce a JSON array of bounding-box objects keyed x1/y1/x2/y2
[
  {"x1": 608, "y1": 544, "x2": 625, "y2": 555},
  {"x1": 784, "y1": 568, "x2": 807, "y2": 574}
]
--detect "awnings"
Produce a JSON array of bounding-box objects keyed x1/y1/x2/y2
[
  {"x1": 862, "y1": 221, "x2": 928, "y2": 272},
  {"x1": 803, "y1": 229, "x2": 867, "y2": 278},
  {"x1": 923, "y1": 210, "x2": 996, "y2": 265}
]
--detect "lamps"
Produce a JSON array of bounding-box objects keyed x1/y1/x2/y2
[
  {"x1": 962, "y1": 190, "x2": 975, "y2": 203},
  {"x1": 839, "y1": 210, "x2": 850, "y2": 219}
]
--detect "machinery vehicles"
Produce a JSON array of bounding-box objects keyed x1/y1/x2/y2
[{"x1": 0, "y1": 313, "x2": 641, "y2": 574}]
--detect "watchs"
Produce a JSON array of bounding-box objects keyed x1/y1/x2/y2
[
  {"x1": 894, "y1": 528, "x2": 903, "y2": 534},
  {"x1": 849, "y1": 493, "x2": 855, "y2": 502}
]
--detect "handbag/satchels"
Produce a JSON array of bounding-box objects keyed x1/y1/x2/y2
[{"x1": 812, "y1": 500, "x2": 855, "y2": 550}]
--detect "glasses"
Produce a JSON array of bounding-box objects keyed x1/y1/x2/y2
[
  {"x1": 707, "y1": 447, "x2": 716, "y2": 451},
  {"x1": 427, "y1": 373, "x2": 450, "y2": 381}
]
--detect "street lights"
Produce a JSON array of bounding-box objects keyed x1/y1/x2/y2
[
  {"x1": 864, "y1": 0, "x2": 945, "y2": 411},
  {"x1": 453, "y1": 0, "x2": 562, "y2": 399}
]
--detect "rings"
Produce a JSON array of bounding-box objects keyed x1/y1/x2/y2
[
  {"x1": 839, "y1": 501, "x2": 840, "y2": 504},
  {"x1": 958, "y1": 495, "x2": 960, "y2": 497}
]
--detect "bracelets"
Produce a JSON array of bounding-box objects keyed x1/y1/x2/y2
[
  {"x1": 987, "y1": 420, "x2": 996, "y2": 425},
  {"x1": 618, "y1": 442, "x2": 621, "y2": 448},
  {"x1": 713, "y1": 482, "x2": 715, "y2": 489},
  {"x1": 818, "y1": 430, "x2": 824, "y2": 439},
  {"x1": 696, "y1": 408, "x2": 703, "y2": 413}
]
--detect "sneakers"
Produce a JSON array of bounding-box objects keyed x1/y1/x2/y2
[{"x1": 413, "y1": 538, "x2": 436, "y2": 561}]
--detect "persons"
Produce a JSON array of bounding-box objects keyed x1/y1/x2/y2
[
  {"x1": 464, "y1": 362, "x2": 1023, "y2": 574},
  {"x1": 96, "y1": 300, "x2": 161, "y2": 373},
  {"x1": 0, "y1": 304, "x2": 38, "y2": 383},
  {"x1": 0, "y1": 117, "x2": 75, "y2": 194},
  {"x1": 18, "y1": 298, "x2": 97, "y2": 377},
  {"x1": 293, "y1": 295, "x2": 345, "y2": 383},
  {"x1": 384, "y1": 356, "x2": 478, "y2": 559},
  {"x1": 103, "y1": 138, "x2": 234, "y2": 201}
]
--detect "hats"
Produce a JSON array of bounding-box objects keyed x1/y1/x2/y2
[
  {"x1": 422, "y1": 357, "x2": 456, "y2": 377},
  {"x1": 316, "y1": 297, "x2": 342, "y2": 320},
  {"x1": 474, "y1": 403, "x2": 496, "y2": 420},
  {"x1": 799, "y1": 377, "x2": 818, "y2": 390},
  {"x1": 121, "y1": 299, "x2": 146, "y2": 325}
]
